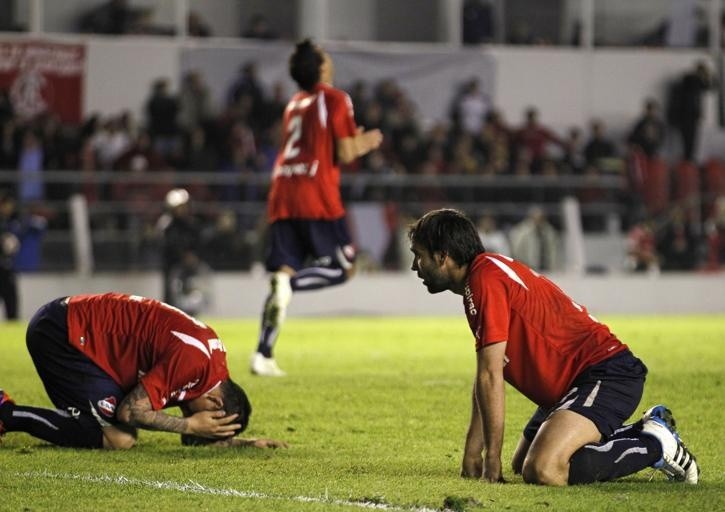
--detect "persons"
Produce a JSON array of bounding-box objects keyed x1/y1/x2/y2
[
  {"x1": 0, "y1": 0, "x2": 724, "y2": 317},
  {"x1": 0, "y1": 293, "x2": 289, "y2": 451},
  {"x1": 252, "y1": 38, "x2": 384, "y2": 373},
  {"x1": 407, "y1": 209, "x2": 699, "y2": 487}
]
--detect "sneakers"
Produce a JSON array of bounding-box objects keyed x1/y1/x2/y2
[
  {"x1": 0, "y1": 390, "x2": 16, "y2": 435},
  {"x1": 251, "y1": 352, "x2": 286, "y2": 376},
  {"x1": 263, "y1": 271, "x2": 293, "y2": 328},
  {"x1": 642, "y1": 405, "x2": 700, "y2": 485}
]
